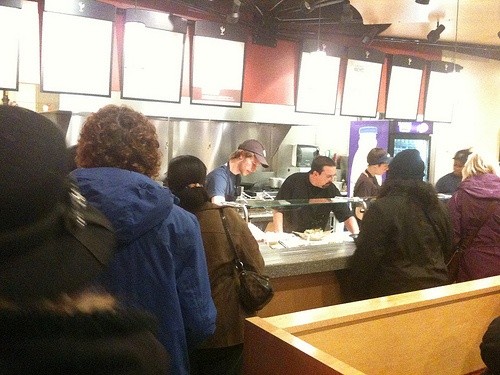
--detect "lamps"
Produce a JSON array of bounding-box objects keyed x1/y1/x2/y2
[
  {"x1": 427, "y1": 14, "x2": 445, "y2": 45},
  {"x1": 227, "y1": 0, "x2": 378, "y2": 45}
]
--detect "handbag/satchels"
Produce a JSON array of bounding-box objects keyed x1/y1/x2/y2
[
  {"x1": 219, "y1": 206, "x2": 272, "y2": 310},
  {"x1": 444, "y1": 241, "x2": 464, "y2": 283}
]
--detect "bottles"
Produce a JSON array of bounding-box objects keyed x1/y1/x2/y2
[{"x1": 349, "y1": 127, "x2": 382, "y2": 211}]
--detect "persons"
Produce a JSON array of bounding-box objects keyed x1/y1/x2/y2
[
  {"x1": 351, "y1": 149, "x2": 458, "y2": 301},
  {"x1": 164, "y1": 155, "x2": 275, "y2": 375},
  {"x1": 344, "y1": 147, "x2": 392, "y2": 232},
  {"x1": 270, "y1": 156, "x2": 360, "y2": 234},
  {"x1": 0, "y1": 104, "x2": 176, "y2": 375},
  {"x1": 206, "y1": 139, "x2": 269, "y2": 206},
  {"x1": 435, "y1": 149, "x2": 472, "y2": 195},
  {"x1": 68, "y1": 105, "x2": 218, "y2": 375},
  {"x1": 448, "y1": 154, "x2": 500, "y2": 283}
]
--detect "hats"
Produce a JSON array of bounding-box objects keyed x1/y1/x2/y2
[
  {"x1": 238, "y1": 140, "x2": 269, "y2": 168},
  {"x1": 453, "y1": 150, "x2": 471, "y2": 160},
  {"x1": 369, "y1": 154, "x2": 393, "y2": 165},
  {"x1": 0, "y1": 105, "x2": 64, "y2": 227}
]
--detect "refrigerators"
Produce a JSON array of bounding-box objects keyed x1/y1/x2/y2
[{"x1": 349, "y1": 121, "x2": 433, "y2": 197}]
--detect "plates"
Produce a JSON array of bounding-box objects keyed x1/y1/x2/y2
[{"x1": 293, "y1": 229, "x2": 333, "y2": 241}]
